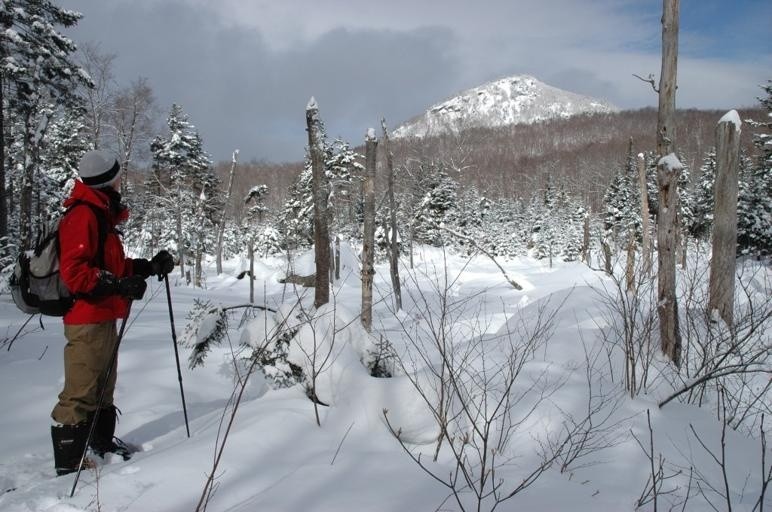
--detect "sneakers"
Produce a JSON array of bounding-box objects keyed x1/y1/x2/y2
[{"x1": 90, "y1": 443, "x2": 131, "y2": 460}]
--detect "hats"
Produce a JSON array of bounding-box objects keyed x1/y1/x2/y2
[{"x1": 78, "y1": 151, "x2": 121, "y2": 189}]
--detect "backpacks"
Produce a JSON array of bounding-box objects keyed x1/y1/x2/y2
[{"x1": 6, "y1": 200, "x2": 124, "y2": 352}]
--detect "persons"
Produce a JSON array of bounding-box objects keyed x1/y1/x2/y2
[{"x1": 50, "y1": 149, "x2": 175, "y2": 478}]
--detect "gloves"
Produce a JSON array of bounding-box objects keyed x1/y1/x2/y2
[
  {"x1": 149, "y1": 250, "x2": 175, "y2": 282},
  {"x1": 119, "y1": 275, "x2": 147, "y2": 303}
]
